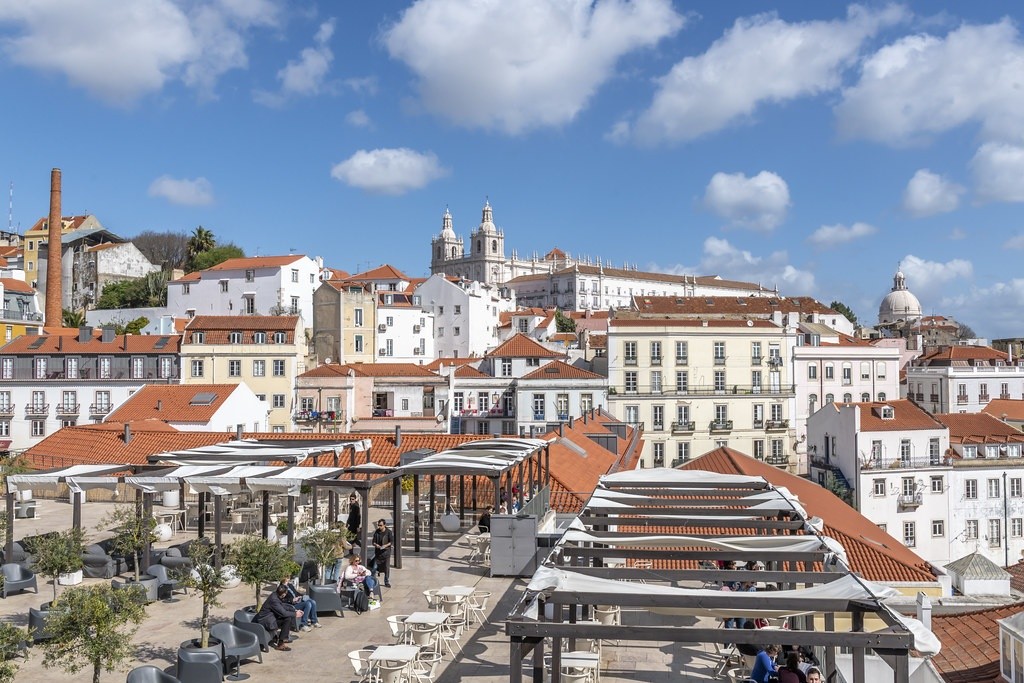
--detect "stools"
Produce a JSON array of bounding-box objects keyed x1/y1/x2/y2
[
  {"x1": 14, "y1": 507, "x2": 21, "y2": 521},
  {"x1": 30, "y1": 506, "x2": 41, "y2": 519}
]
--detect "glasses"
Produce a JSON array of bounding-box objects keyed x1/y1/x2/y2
[
  {"x1": 350, "y1": 499, "x2": 354, "y2": 500},
  {"x1": 378, "y1": 525, "x2": 384, "y2": 527},
  {"x1": 354, "y1": 560, "x2": 360, "y2": 562}
]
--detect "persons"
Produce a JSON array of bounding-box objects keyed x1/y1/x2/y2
[
  {"x1": 479, "y1": 506, "x2": 493, "y2": 534},
  {"x1": 716, "y1": 548, "x2": 822, "y2": 683},
  {"x1": 280, "y1": 575, "x2": 322, "y2": 632},
  {"x1": 330, "y1": 521, "x2": 352, "y2": 582},
  {"x1": 251, "y1": 585, "x2": 304, "y2": 651},
  {"x1": 492, "y1": 484, "x2": 527, "y2": 514},
  {"x1": 372, "y1": 519, "x2": 393, "y2": 588},
  {"x1": 344, "y1": 494, "x2": 361, "y2": 558},
  {"x1": 533, "y1": 480, "x2": 539, "y2": 494},
  {"x1": 345, "y1": 555, "x2": 377, "y2": 598}
]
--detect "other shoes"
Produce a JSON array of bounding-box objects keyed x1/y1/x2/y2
[
  {"x1": 311, "y1": 622, "x2": 322, "y2": 628},
  {"x1": 300, "y1": 626, "x2": 311, "y2": 632},
  {"x1": 282, "y1": 638, "x2": 292, "y2": 643},
  {"x1": 385, "y1": 580, "x2": 391, "y2": 588},
  {"x1": 726, "y1": 658, "x2": 730, "y2": 666},
  {"x1": 345, "y1": 553, "x2": 354, "y2": 558},
  {"x1": 276, "y1": 645, "x2": 291, "y2": 651}
]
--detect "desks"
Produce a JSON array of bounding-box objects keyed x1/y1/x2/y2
[
  {"x1": 561, "y1": 654, "x2": 599, "y2": 683},
  {"x1": 477, "y1": 533, "x2": 491, "y2": 565},
  {"x1": 231, "y1": 508, "x2": 258, "y2": 535},
  {"x1": 562, "y1": 622, "x2": 602, "y2": 662},
  {"x1": 187, "y1": 503, "x2": 215, "y2": 527},
  {"x1": 278, "y1": 512, "x2": 303, "y2": 536},
  {"x1": 305, "y1": 505, "x2": 328, "y2": 529},
  {"x1": 369, "y1": 646, "x2": 421, "y2": 683},
  {"x1": 436, "y1": 587, "x2": 475, "y2": 631},
  {"x1": 160, "y1": 509, "x2": 186, "y2": 536},
  {"x1": 403, "y1": 612, "x2": 450, "y2": 662},
  {"x1": 250, "y1": 502, "x2": 275, "y2": 514}
]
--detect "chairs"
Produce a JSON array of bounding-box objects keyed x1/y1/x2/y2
[
  {"x1": 0, "y1": 490, "x2": 652, "y2": 683},
  {"x1": 699, "y1": 552, "x2": 841, "y2": 683}
]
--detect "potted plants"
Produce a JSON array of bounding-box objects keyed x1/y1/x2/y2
[{"x1": 57, "y1": 529, "x2": 86, "y2": 586}]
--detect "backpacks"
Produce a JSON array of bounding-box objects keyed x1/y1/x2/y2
[{"x1": 353, "y1": 591, "x2": 368, "y2": 615}]
